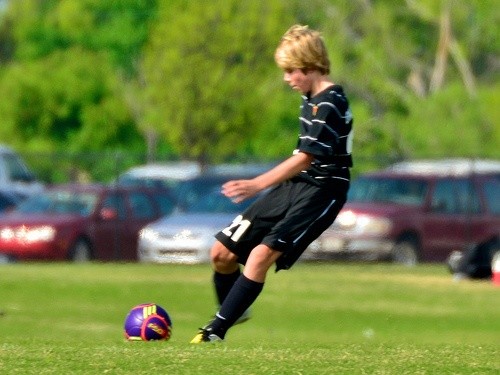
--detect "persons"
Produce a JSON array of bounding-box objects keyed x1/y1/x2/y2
[
  {"x1": 188, "y1": 23, "x2": 356, "y2": 345},
  {"x1": 446, "y1": 231, "x2": 500, "y2": 286}
]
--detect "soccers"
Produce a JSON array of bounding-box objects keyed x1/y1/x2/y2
[{"x1": 124, "y1": 303, "x2": 173, "y2": 342}]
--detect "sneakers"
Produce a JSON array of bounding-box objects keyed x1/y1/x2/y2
[
  {"x1": 234, "y1": 309, "x2": 252, "y2": 327},
  {"x1": 190, "y1": 326, "x2": 224, "y2": 343}
]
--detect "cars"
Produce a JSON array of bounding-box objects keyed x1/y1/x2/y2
[
  {"x1": 0, "y1": 185, "x2": 162, "y2": 264},
  {"x1": 0, "y1": 143, "x2": 44, "y2": 197},
  {"x1": 137, "y1": 175, "x2": 274, "y2": 263},
  {"x1": 303, "y1": 172, "x2": 500, "y2": 273}
]
